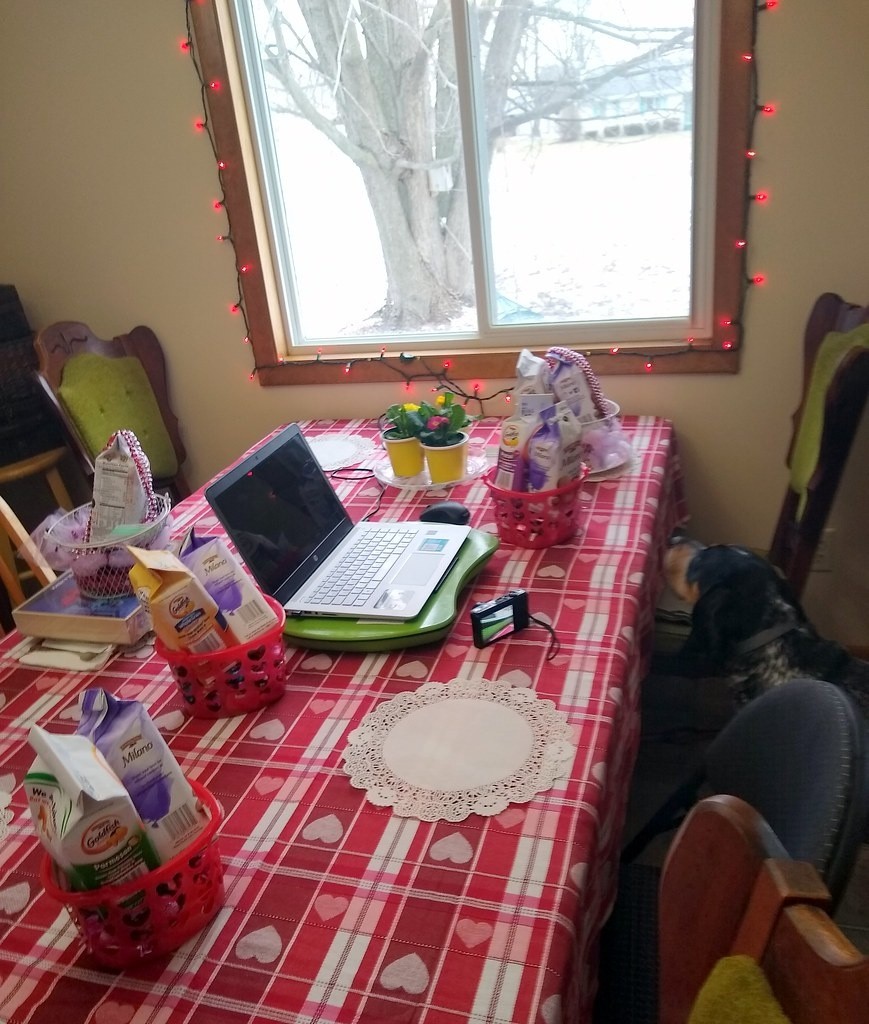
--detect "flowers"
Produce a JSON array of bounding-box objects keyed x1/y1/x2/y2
[{"x1": 384, "y1": 390, "x2": 475, "y2": 447}]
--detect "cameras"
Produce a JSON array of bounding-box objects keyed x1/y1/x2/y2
[{"x1": 469, "y1": 588, "x2": 530, "y2": 649}]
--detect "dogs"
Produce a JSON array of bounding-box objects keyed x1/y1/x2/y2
[{"x1": 654, "y1": 539, "x2": 869, "y2": 729}]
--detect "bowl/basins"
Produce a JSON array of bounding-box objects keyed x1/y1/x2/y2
[{"x1": 580, "y1": 399, "x2": 620, "y2": 470}]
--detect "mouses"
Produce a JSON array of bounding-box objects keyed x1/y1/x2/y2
[{"x1": 420, "y1": 501, "x2": 470, "y2": 526}]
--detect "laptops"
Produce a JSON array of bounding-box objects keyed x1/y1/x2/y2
[{"x1": 203, "y1": 422, "x2": 472, "y2": 619}]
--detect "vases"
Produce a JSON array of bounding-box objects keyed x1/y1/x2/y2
[{"x1": 380, "y1": 428, "x2": 469, "y2": 483}]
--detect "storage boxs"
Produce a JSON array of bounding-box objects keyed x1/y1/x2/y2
[{"x1": 580, "y1": 398, "x2": 620, "y2": 470}]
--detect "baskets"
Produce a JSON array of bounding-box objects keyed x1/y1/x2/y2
[
  {"x1": 156, "y1": 589, "x2": 288, "y2": 714},
  {"x1": 36, "y1": 781, "x2": 228, "y2": 965},
  {"x1": 483, "y1": 465, "x2": 588, "y2": 551}
]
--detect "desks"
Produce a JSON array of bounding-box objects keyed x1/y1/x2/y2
[{"x1": 0, "y1": 414, "x2": 674, "y2": 1024}]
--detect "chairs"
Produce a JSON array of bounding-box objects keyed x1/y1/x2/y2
[
  {"x1": 31, "y1": 320, "x2": 190, "y2": 506},
  {"x1": 591, "y1": 678, "x2": 869, "y2": 1024},
  {"x1": 651, "y1": 293, "x2": 869, "y2": 659}
]
